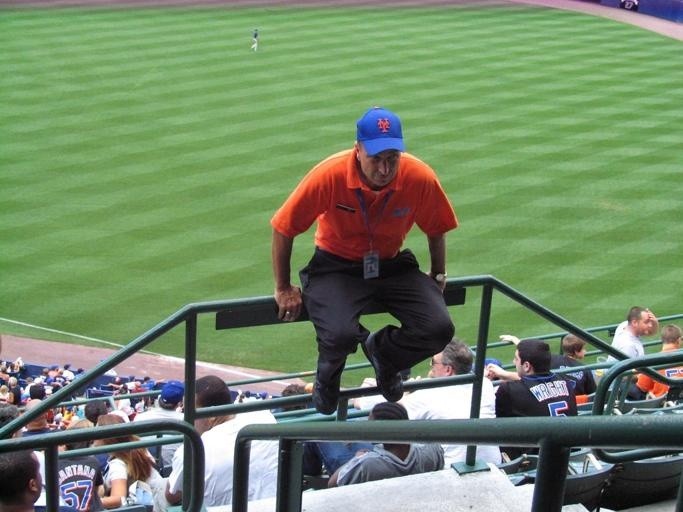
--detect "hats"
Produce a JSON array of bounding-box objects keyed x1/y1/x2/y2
[
  {"x1": 357, "y1": 107, "x2": 405, "y2": 156},
  {"x1": 158, "y1": 380, "x2": 185, "y2": 408}
]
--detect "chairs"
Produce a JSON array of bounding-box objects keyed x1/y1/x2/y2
[{"x1": 0, "y1": 360, "x2": 682, "y2": 511}]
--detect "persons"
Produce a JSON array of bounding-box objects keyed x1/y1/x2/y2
[
  {"x1": 250, "y1": 29, "x2": 259, "y2": 51},
  {"x1": 270, "y1": 106, "x2": 456, "y2": 416},
  {"x1": 0, "y1": 305, "x2": 682, "y2": 510}
]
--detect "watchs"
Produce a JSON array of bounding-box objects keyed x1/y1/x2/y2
[{"x1": 428, "y1": 270, "x2": 447, "y2": 284}]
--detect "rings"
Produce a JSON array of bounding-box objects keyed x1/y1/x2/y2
[{"x1": 285, "y1": 312, "x2": 291, "y2": 317}]
